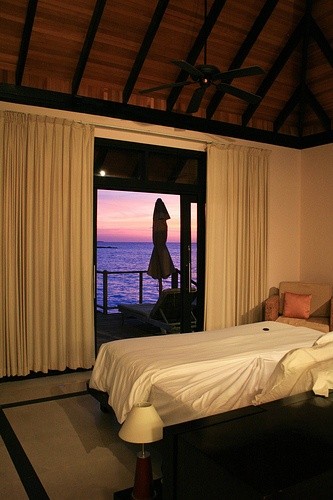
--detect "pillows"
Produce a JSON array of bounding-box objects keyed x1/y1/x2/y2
[
  {"x1": 281, "y1": 291, "x2": 311, "y2": 320},
  {"x1": 250, "y1": 330, "x2": 333, "y2": 408}
]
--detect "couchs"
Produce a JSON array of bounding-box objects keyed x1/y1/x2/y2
[{"x1": 264, "y1": 281, "x2": 333, "y2": 334}]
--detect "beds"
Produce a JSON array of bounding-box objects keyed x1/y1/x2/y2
[{"x1": 87, "y1": 320, "x2": 327, "y2": 428}]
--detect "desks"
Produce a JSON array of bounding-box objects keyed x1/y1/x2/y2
[{"x1": 158, "y1": 388, "x2": 333, "y2": 500}]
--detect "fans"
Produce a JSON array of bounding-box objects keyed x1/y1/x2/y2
[{"x1": 136, "y1": 0, "x2": 263, "y2": 113}]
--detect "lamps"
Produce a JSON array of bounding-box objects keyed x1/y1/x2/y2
[{"x1": 117, "y1": 402, "x2": 166, "y2": 500}]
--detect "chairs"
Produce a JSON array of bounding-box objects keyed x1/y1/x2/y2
[{"x1": 117, "y1": 287, "x2": 197, "y2": 336}]
